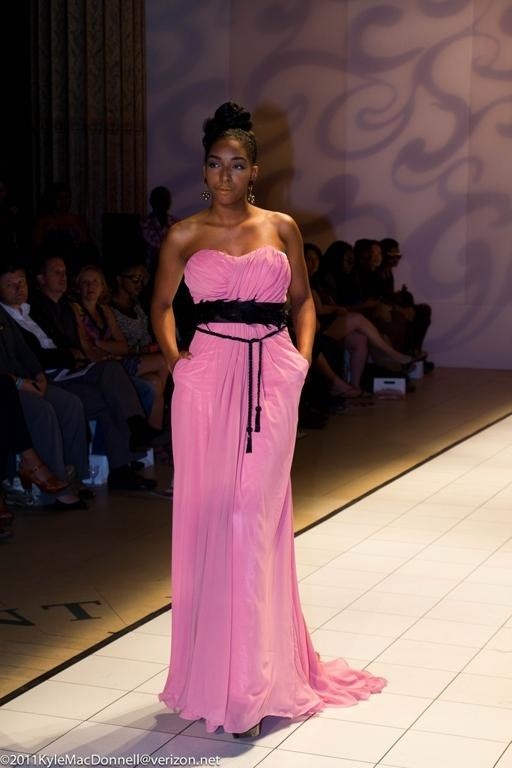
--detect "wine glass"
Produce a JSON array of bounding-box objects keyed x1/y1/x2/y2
[{"x1": 86, "y1": 464, "x2": 100, "y2": 488}]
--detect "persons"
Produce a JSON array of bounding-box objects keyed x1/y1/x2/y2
[
  {"x1": 1, "y1": 182, "x2": 439, "y2": 541},
  {"x1": 152, "y1": 101, "x2": 389, "y2": 738}
]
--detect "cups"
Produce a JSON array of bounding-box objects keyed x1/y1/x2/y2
[{"x1": 409, "y1": 359, "x2": 424, "y2": 379}]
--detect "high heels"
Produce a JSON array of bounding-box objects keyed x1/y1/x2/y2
[{"x1": 19, "y1": 455, "x2": 69, "y2": 493}]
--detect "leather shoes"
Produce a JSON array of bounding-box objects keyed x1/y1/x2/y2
[
  {"x1": 107, "y1": 466, "x2": 156, "y2": 490},
  {"x1": 130, "y1": 424, "x2": 169, "y2": 453}
]
